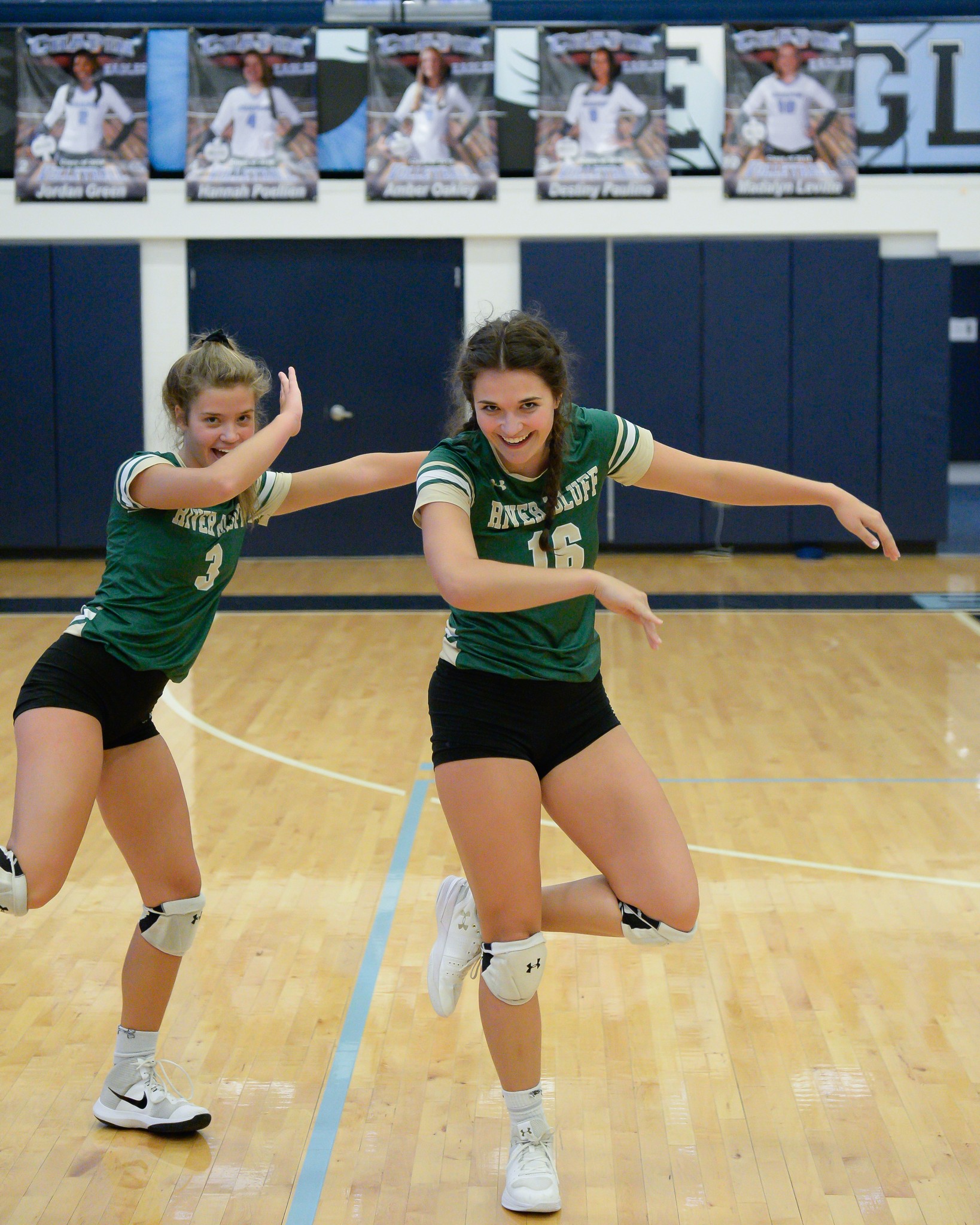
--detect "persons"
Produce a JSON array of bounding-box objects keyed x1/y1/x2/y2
[
  {"x1": 377, "y1": 44, "x2": 481, "y2": 157},
  {"x1": 558, "y1": 48, "x2": 652, "y2": 144},
  {"x1": 0, "y1": 331, "x2": 432, "y2": 1132},
  {"x1": 406, "y1": 309, "x2": 900, "y2": 1213},
  {"x1": 23, "y1": 48, "x2": 137, "y2": 154},
  {"x1": 193, "y1": 49, "x2": 304, "y2": 162},
  {"x1": 732, "y1": 42, "x2": 839, "y2": 164}
]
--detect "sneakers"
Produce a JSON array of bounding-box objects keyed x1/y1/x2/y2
[
  {"x1": 91, "y1": 1068, "x2": 212, "y2": 1139},
  {"x1": 500, "y1": 1118, "x2": 562, "y2": 1215},
  {"x1": 426, "y1": 876, "x2": 483, "y2": 1017}
]
